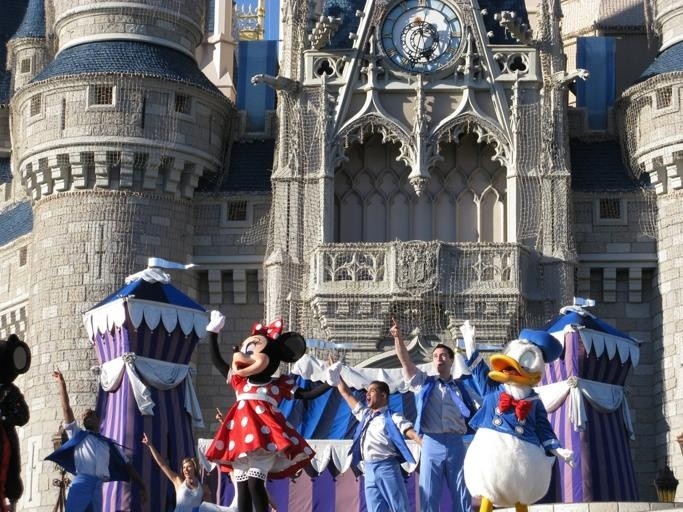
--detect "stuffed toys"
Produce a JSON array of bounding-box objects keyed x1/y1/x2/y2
[
  {"x1": 459, "y1": 319, "x2": 579, "y2": 511},
  {"x1": 206, "y1": 310, "x2": 342, "y2": 512},
  {"x1": 0, "y1": 334, "x2": 32, "y2": 511}
]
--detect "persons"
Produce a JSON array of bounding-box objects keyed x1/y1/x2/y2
[
  {"x1": 389, "y1": 316, "x2": 478, "y2": 511},
  {"x1": 326, "y1": 354, "x2": 422, "y2": 512},
  {"x1": 52, "y1": 366, "x2": 151, "y2": 510},
  {"x1": 143, "y1": 433, "x2": 213, "y2": 511},
  {"x1": 216, "y1": 408, "x2": 277, "y2": 510}
]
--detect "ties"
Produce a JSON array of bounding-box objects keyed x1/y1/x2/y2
[
  {"x1": 347, "y1": 413, "x2": 376, "y2": 458},
  {"x1": 439, "y1": 379, "x2": 471, "y2": 418},
  {"x1": 94, "y1": 434, "x2": 136, "y2": 452}
]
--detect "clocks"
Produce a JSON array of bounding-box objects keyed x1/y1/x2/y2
[{"x1": 381, "y1": 0, "x2": 462, "y2": 73}]
